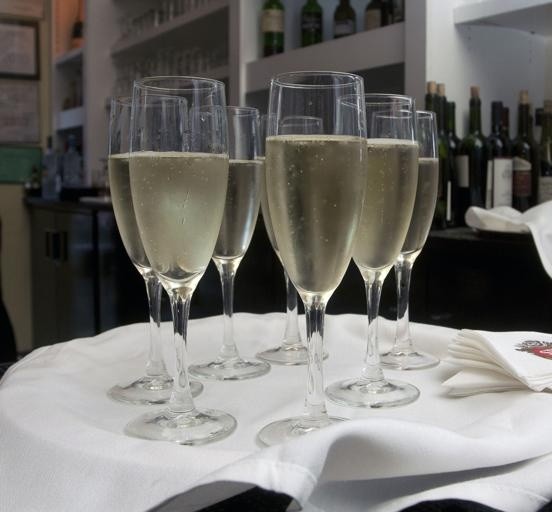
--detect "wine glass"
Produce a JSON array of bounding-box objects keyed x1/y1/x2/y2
[
  {"x1": 253, "y1": 70, "x2": 441, "y2": 455},
  {"x1": 107, "y1": 72, "x2": 273, "y2": 448}
]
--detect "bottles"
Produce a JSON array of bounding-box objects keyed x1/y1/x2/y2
[
  {"x1": 260, "y1": 1, "x2": 406, "y2": 58},
  {"x1": 426, "y1": 78, "x2": 551, "y2": 227},
  {"x1": 68, "y1": 1, "x2": 84, "y2": 50},
  {"x1": 23, "y1": 132, "x2": 84, "y2": 197}
]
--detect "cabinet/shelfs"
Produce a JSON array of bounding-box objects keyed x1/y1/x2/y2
[{"x1": 42, "y1": 0, "x2": 551, "y2": 194}]
